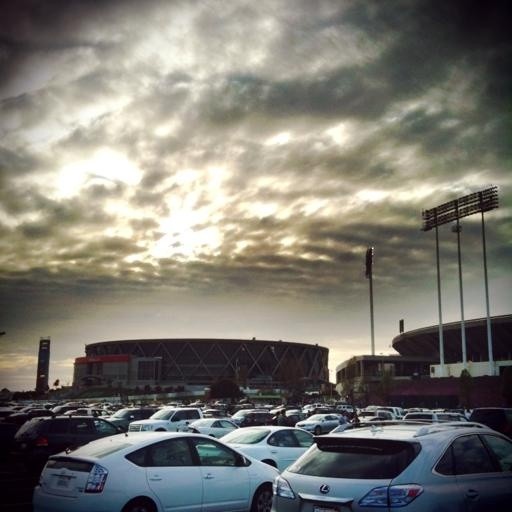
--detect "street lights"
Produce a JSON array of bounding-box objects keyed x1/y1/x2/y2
[
  {"x1": 397, "y1": 319, "x2": 405, "y2": 335},
  {"x1": 365, "y1": 244, "x2": 378, "y2": 356}
]
